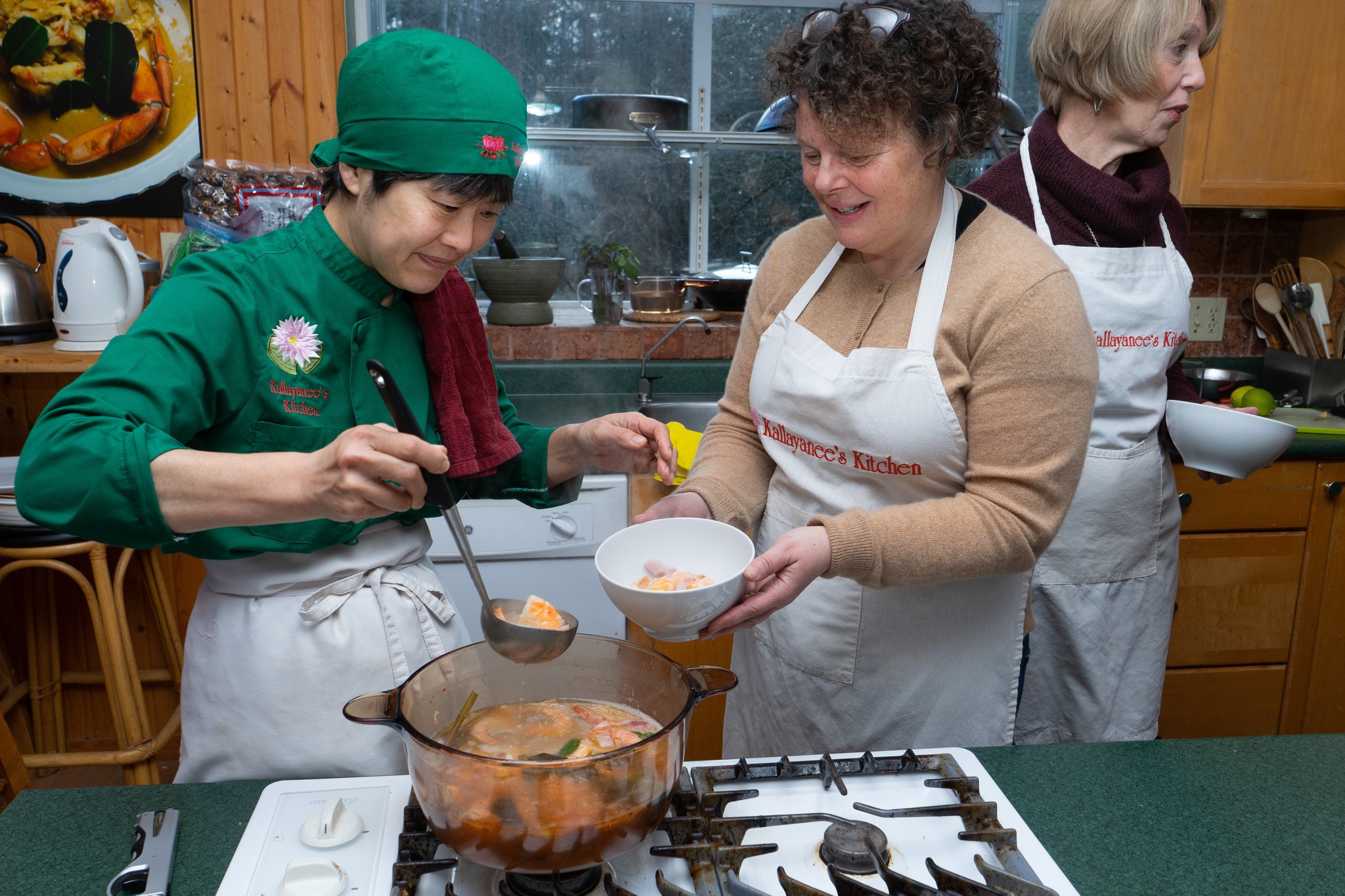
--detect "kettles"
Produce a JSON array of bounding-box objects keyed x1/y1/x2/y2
[
  {"x1": 0, "y1": 214, "x2": 58, "y2": 345},
  {"x1": 53, "y1": 217, "x2": 145, "y2": 351}
]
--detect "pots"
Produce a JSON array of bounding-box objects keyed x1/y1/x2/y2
[
  {"x1": 677, "y1": 252, "x2": 759, "y2": 311},
  {"x1": 983, "y1": 91, "x2": 1027, "y2": 161},
  {"x1": 572, "y1": 94, "x2": 689, "y2": 155},
  {"x1": 343, "y1": 633, "x2": 739, "y2": 873},
  {"x1": 626, "y1": 276, "x2": 720, "y2": 314}
]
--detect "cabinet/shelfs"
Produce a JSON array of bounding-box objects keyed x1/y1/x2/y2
[
  {"x1": 1162, "y1": 0, "x2": 1345, "y2": 210},
  {"x1": 1156, "y1": 460, "x2": 1345, "y2": 738},
  {"x1": 351, "y1": 0, "x2": 1026, "y2": 324}
]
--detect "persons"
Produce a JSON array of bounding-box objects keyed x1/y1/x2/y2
[
  {"x1": 632, "y1": 0, "x2": 1098, "y2": 762},
  {"x1": 957, "y1": 0, "x2": 1276, "y2": 751},
  {"x1": 14, "y1": 25, "x2": 678, "y2": 785}
]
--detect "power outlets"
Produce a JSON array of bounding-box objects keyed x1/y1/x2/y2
[{"x1": 1188, "y1": 297, "x2": 1227, "y2": 341}]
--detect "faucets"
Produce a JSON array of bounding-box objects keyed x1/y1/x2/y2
[{"x1": 634, "y1": 316, "x2": 711, "y2": 404}]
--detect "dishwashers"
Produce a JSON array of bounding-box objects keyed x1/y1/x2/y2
[{"x1": 425, "y1": 474, "x2": 628, "y2": 644}]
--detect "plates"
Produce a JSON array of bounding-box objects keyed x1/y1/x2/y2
[{"x1": 0, "y1": 0, "x2": 200, "y2": 203}]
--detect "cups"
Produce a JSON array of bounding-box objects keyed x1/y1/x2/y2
[{"x1": 577, "y1": 269, "x2": 627, "y2": 324}]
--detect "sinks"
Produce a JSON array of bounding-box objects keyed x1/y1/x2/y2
[{"x1": 629, "y1": 400, "x2": 720, "y2": 433}]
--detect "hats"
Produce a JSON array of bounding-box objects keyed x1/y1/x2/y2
[{"x1": 310, "y1": 30, "x2": 528, "y2": 180}]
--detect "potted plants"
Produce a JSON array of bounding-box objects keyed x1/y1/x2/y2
[{"x1": 576, "y1": 243, "x2": 641, "y2": 327}]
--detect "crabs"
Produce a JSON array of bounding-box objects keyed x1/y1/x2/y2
[{"x1": 1, "y1": 0, "x2": 177, "y2": 171}]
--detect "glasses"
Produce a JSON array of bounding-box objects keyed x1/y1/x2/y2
[{"x1": 802, "y1": 6, "x2": 910, "y2": 50}]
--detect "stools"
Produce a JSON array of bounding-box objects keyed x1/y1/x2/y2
[{"x1": 0, "y1": 523, "x2": 184, "y2": 790}]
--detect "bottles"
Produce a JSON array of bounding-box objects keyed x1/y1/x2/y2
[{"x1": 139, "y1": 259, "x2": 161, "y2": 311}]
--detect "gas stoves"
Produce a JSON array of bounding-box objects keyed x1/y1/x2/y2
[{"x1": 214, "y1": 746, "x2": 1081, "y2": 896}]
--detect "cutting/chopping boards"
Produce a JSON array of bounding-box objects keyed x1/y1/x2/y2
[{"x1": 1265, "y1": 408, "x2": 1345, "y2": 435}]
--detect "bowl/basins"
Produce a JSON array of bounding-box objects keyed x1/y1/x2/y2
[
  {"x1": 1182, "y1": 368, "x2": 1258, "y2": 403},
  {"x1": 593, "y1": 517, "x2": 755, "y2": 642},
  {"x1": 0, "y1": 456, "x2": 38, "y2": 526},
  {"x1": 1165, "y1": 399, "x2": 1298, "y2": 480}
]
